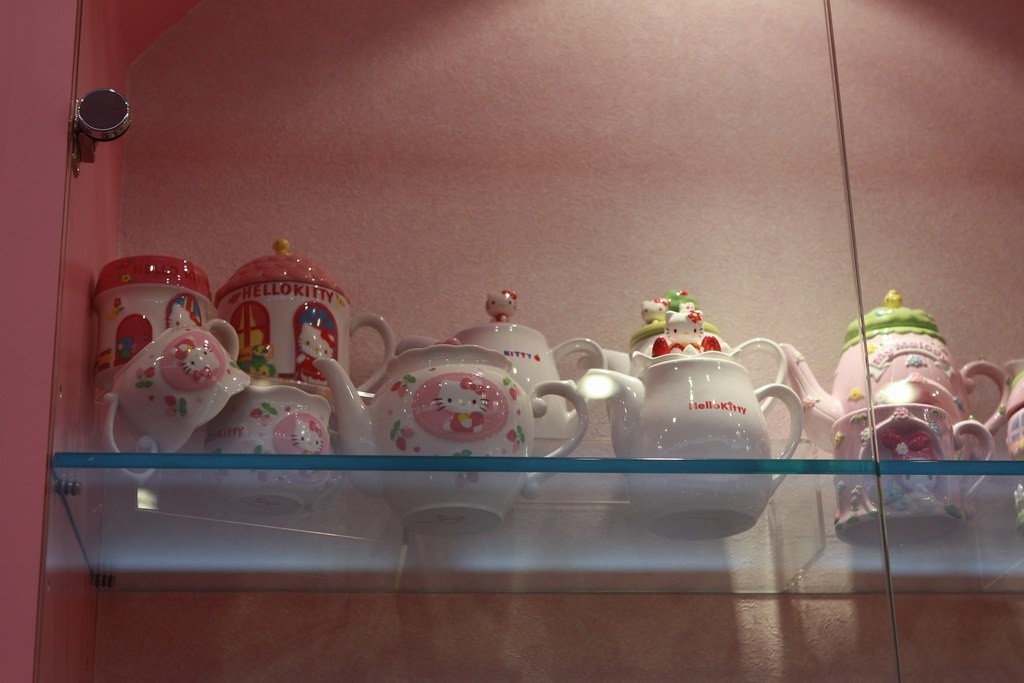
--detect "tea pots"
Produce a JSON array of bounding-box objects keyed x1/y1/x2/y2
[{"x1": 313, "y1": 324, "x2": 1024, "y2": 543}]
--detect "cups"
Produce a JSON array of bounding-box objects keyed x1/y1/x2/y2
[
  {"x1": 831, "y1": 404, "x2": 994, "y2": 544},
  {"x1": 93, "y1": 238, "x2": 395, "y2": 517}
]
